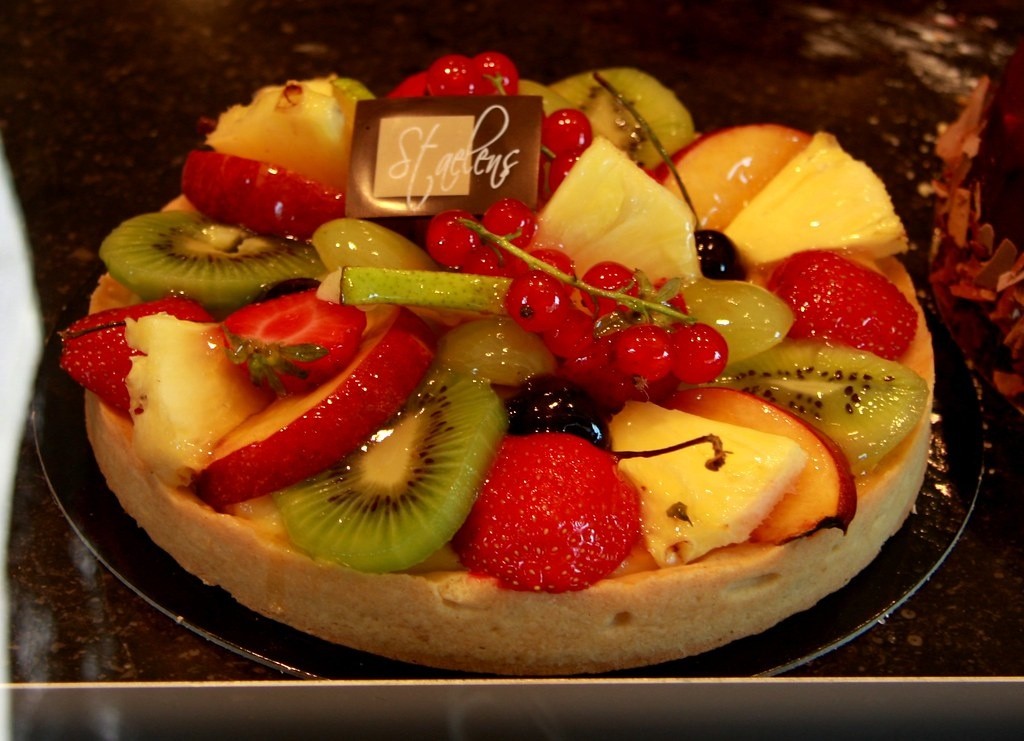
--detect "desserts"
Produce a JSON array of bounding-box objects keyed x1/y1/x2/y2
[{"x1": 62, "y1": 52, "x2": 938, "y2": 675}]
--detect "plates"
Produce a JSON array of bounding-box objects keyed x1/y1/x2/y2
[{"x1": 31, "y1": 256, "x2": 990, "y2": 679}]
